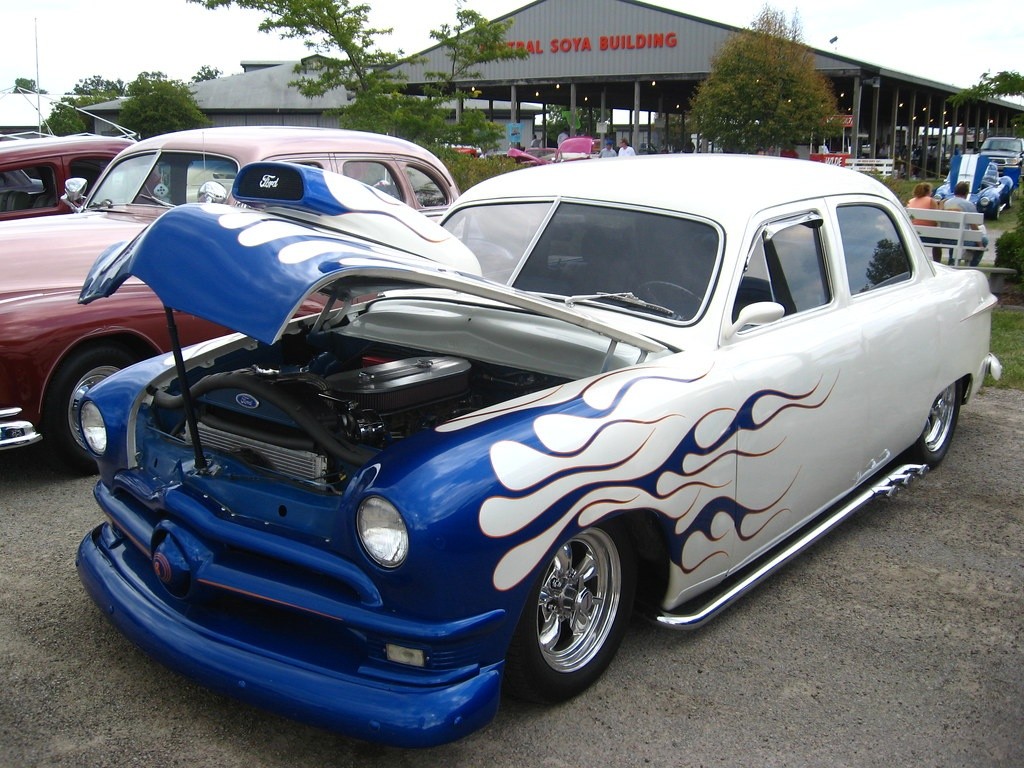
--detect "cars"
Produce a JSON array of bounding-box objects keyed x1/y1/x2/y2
[
  {"x1": 1, "y1": 136, "x2": 171, "y2": 220},
  {"x1": 933, "y1": 153, "x2": 1014, "y2": 221},
  {"x1": 77, "y1": 150, "x2": 1001, "y2": 754},
  {"x1": 444, "y1": 137, "x2": 602, "y2": 168},
  {"x1": 1, "y1": 123, "x2": 513, "y2": 475}
]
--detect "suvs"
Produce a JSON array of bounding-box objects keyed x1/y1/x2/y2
[{"x1": 977, "y1": 137, "x2": 1024, "y2": 171}]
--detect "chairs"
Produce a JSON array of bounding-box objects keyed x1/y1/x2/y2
[
  {"x1": 32, "y1": 194, "x2": 50, "y2": 208},
  {"x1": 574, "y1": 224, "x2": 629, "y2": 295},
  {"x1": 732, "y1": 240, "x2": 819, "y2": 322},
  {"x1": 0, "y1": 190, "x2": 30, "y2": 211}
]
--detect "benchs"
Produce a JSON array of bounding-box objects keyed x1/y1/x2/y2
[
  {"x1": 907, "y1": 207, "x2": 989, "y2": 266},
  {"x1": 951, "y1": 265, "x2": 1017, "y2": 294},
  {"x1": 844, "y1": 158, "x2": 893, "y2": 179}
]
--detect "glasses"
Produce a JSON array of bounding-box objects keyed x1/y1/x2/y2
[{"x1": 619, "y1": 142, "x2": 624, "y2": 144}]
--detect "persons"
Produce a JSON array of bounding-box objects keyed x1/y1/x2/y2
[
  {"x1": 672, "y1": 138, "x2": 695, "y2": 153},
  {"x1": 906, "y1": 182, "x2": 942, "y2": 262},
  {"x1": 531, "y1": 127, "x2": 569, "y2": 150},
  {"x1": 941, "y1": 180, "x2": 989, "y2": 267},
  {"x1": 598, "y1": 140, "x2": 617, "y2": 158},
  {"x1": 877, "y1": 142, "x2": 961, "y2": 172},
  {"x1": 618, "y1": 139, "x2": 635, "y2": 156}
]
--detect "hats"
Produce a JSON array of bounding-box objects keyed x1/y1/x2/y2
[
  {"x1": 757, "y1": 147, "x2": 767, "y2": 152},
  {"x1": 606, "y1": 141, "x2": 612, "y2": 145}
]
